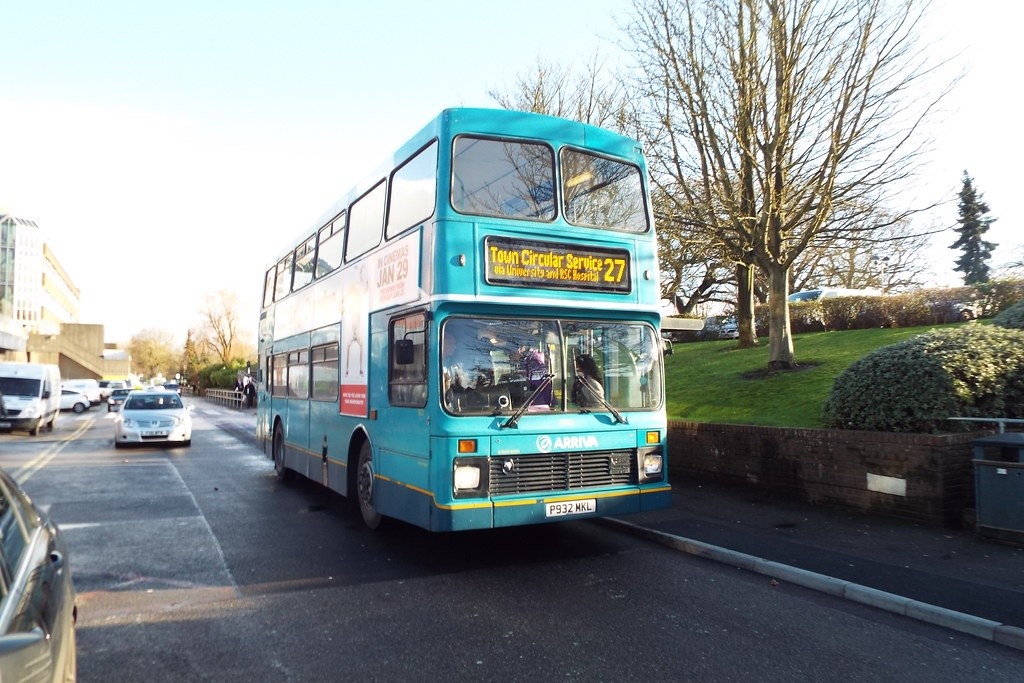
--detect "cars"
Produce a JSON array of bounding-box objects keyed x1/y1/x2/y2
[
  {"x1": 953, "y1": 298, "x2": 995, "y2": 321},
  {"x1": 111, "y1": 387, "x2": 194, "y2": 448},
  {"x1": 0, "y1": 467, "x2": 79, "y2": 683},
  {"x1": 706, "y1": 316, "x2": 738, "y2": 338},
  {"x1": 60, "y1": 388, "x2": 92, "y2": 413},
  {"x1": 164, "y1": 383, "x2": 181, "y2": 395},
  {"x1": 107, "y1": 389, "x2": 131, "y2": 412}
]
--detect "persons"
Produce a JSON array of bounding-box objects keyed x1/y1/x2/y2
[
  {"x1": 244, "y1": 380, "x2": 256, "y2": 408},
  {"x1": 442, "y1": 332, "x2": 557, "y2": 406},
  {"x1": 235, "y1": 378, "x2": 244, "y2": 402},
  {"x1": 571, "y1": 354, "x2": 605, "y2": 408}
]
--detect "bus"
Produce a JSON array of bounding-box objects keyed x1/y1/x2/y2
[{"x1": 256, "y1": 109, "x2": 676, "y2": 539}]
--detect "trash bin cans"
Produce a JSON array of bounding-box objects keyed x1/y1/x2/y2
[{"x1": 971, "y1": 433, "x2": 1024, "y2": 545}]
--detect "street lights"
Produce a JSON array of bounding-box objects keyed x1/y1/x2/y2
[{"x1": 880, "y1": 257, "x2": 890, "y2": 294}]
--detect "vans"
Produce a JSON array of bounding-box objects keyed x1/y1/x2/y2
[
  {"x1": 97, "y1": 380, "x2": 133, "y2": 401},
  {"x1": 61, "y1": 379, "x2": 101, "y2": 405},
  {"x1": 789, "y1": 289, "x2": 881, "y2": 323},
  {"x1": 1, "y1": 362, "x2": 62, "y2": 436}
]
